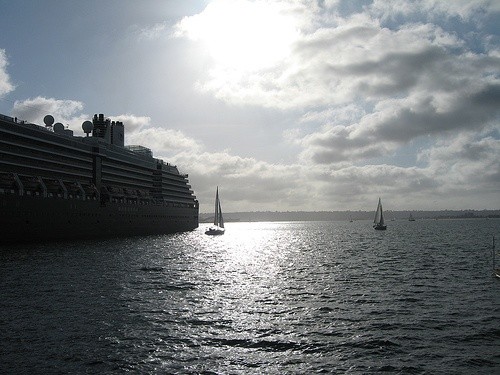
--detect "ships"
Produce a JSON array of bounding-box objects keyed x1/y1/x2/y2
[{"x1": 0, "y1": 114, "x2": 198, "y2": 251}]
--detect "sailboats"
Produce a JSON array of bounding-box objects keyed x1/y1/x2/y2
[
  {"x1": 350, "y1": 213, "x2": 416, "y2": 226},
  {"x1": 203, "y1": 186, "x2": 226, "y2": 236},
  {"x1": 373, "y1": 198, "x2": 386, "y2": 229}
]
